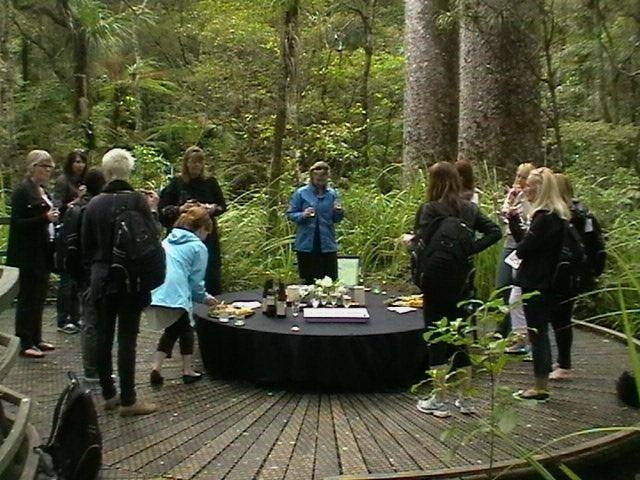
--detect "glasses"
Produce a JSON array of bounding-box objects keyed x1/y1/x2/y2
[{"x1": 310, "y1": 167, "x2": 328, "y2": 173}]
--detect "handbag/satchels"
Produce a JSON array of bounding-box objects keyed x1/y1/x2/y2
[{"x1": 33, "y1": 371, "x2": 102, "y2": 480}]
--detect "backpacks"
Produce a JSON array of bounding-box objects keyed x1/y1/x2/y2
[
  {"x1": 102, "y1": 191, "x2": 166, "y2": 299},
  {"x1": 530, "y1": 208, "x2": 584, "y2": 304},
  {"x1": 407, "y1": 200, "x2": 475, "y2": 296},
  {"x1": 49, "y1": 207, "x2": 83, "y2": 276},
  {"x1": 571, "y1": 210, "x2": 606, "y2": 285}
]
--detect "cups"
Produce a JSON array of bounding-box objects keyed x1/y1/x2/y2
[
  {"x1": 219, "y1": 307, "x2": 229, "y2": 323},
  {"x1": 233, "y1": 309, "x2": 245, "y2": 326},
  {"x1": 292, "y1": 299, "x2": 299, "y2": 318}
]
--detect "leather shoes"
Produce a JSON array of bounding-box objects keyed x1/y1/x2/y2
[
  {"x1": 150, "y1": 370, "x2": 163, "y2": 385},
  {"x1": 183, "y1": 371, "x2": 203, "y2": 384}
]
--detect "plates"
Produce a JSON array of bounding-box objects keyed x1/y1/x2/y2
[{"x1": 207, "y1": 311, "x2": 255, "y2": 318}]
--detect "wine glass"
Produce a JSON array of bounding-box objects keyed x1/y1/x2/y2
[{"x1": 310, "y1": 293, "x2": 351, "y2": 308}]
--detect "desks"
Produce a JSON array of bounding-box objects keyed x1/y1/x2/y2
[{"x1": 192, "y1": 286, "x2": 455, "y2": 395}]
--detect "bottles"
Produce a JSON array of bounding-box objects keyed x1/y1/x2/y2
[
  {"x1": 353, "y1": 286, "x2": 364, "y2": 306},
  {"x1": 288, "y1": 286, "x2": 300, "y2": 302},
  {"x1": 371, "y1": 276, "x2": 387, "y2": 295},
  {"x1": 262, "y1": 279, "x2": 287, "y2": 319}
]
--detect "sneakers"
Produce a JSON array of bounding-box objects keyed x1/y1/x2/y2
[
  {"x1": 416, "y1": 393, "x2": 451, "y2": 418},
  {"x1": 490, "y1": 331, "x2": 574, "y2": 404},
  {"x1": 83, "y1": 372, "x2": 117, "y2": 382},
  {"x1": 119, "y1": 399, "x2": 157, "y2": 416},
  {"x1": 455, "y1": 396, "x2": 476, "y2": 415},
  {"x1": 105, "y1": 391, "x2": 122, "y2": 410},
  {"x1": 22, "y1": 316, "x2": 86, "y2": 358}
]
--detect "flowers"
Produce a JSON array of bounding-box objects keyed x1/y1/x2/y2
[{"x1": 297, "y1": 276, "x2": 353, "y2": 309}]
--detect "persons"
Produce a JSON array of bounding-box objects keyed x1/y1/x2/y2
[{"x1": 285, "y1": 160, "x2": 346, "y2": 284}]
